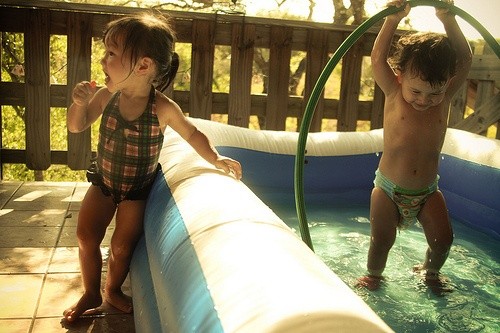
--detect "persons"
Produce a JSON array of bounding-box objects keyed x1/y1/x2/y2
[
  {"x1": 62, "y1": 15, "x2": 243, "y2": 321},
  {"x1": 366, "y1": 0, "x2": 473, "y2": 278}
]
